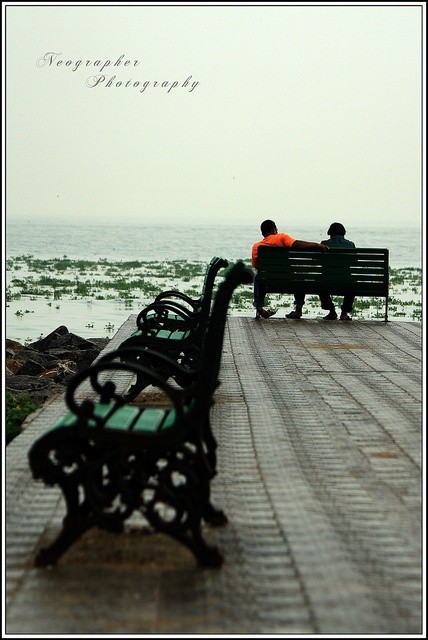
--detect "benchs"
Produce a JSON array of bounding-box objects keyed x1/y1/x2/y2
[
  {"x1": 256, "y1": 248, "x2": 389, "y2": 319},
  {"x1": 26, "y1": 258, "x2": 257, "y2": 568},
  {"x1": 119, "y1": 327, "x2": 212, "y2": 403},
  {"x1": 125, "y1": 254, "x2": 227, "y2": 323}
]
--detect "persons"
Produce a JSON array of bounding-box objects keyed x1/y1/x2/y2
[
  {"x1": 251, "y1": 219, "x2": 329, "y2": 320},
  {"x1": 322, "y1": 223, "x2": 356, "y2": 324}
]
